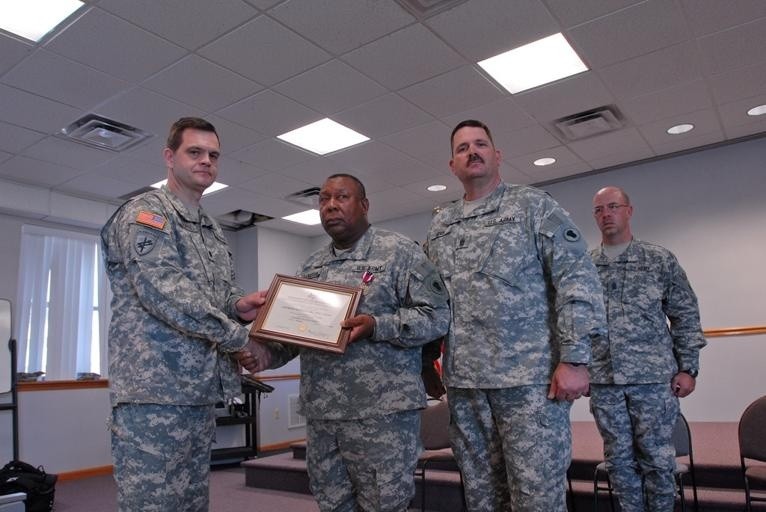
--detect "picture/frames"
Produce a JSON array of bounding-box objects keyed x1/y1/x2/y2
[{"x1": 248, "y1": 272, "x2": 363, "y2": 356}]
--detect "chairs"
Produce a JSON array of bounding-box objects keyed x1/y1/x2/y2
[
  {"x1": 411, "y1": 396, "x2": 466, "y2": 511},
  {"x1": 593, "y1": 412, "x2": 698, "y2": 511},
  {"x1": 737, "y1": 395, "x2": 766, "y2": 512}
]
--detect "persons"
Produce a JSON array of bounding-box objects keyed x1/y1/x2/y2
[
  {"x1": 236, "y1": 171, "x2": 452, "y2": 511},
  {"x1": 100, "y1": 116, "x2": 272, "y2": 511},
  {"x1": 419, "y1": 120, "x2": 601, "y2": 512},
  {"x1": 582, "y1": 185, "x2": 705, "y2": 510}
]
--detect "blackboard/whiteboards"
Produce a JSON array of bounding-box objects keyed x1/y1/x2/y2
[{"x1": 0, "y1": 299, "x2": 13, "y2": 396}]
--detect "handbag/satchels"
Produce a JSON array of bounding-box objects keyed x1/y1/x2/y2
[{"x1": 0, "y1": 459, "x2": 58, "y2": 512}]
